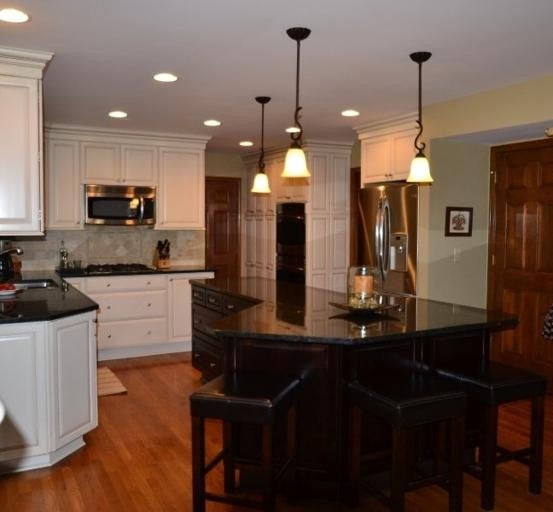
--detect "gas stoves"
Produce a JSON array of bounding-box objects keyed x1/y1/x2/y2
[{"x1": 86, "y1": 263, "x2": 151, "y2": 272}]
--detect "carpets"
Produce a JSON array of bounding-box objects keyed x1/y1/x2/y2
[{"x1": 98, "y1": 365, "x2": 127, "y2": 398}]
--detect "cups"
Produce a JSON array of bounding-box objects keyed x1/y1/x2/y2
[
  {"x1": 347, "y1": 266, "x2": 383, "y2": 309},
  {"x1": 73, "y1": 259, "x2": 82, "y2": 272},
  {"x1": 350, "y1": 326, "x2": 379, "y2": 337}
]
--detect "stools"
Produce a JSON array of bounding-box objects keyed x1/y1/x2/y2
[
  {"x1": 434, "y1": 359, "x2": 546, "y2": 511},
  {"x1": 342, "y1": 366, "x2": 467, "y2": 511},
  {"x1": 190, "y1": 362, "x2": 313, "y2": 512}
]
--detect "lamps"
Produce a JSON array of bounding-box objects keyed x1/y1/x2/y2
[
  {"x1": 405, "y1": 51, "x2": 434, "y2": 183},
  {"x1": 250, "y1": 96, "x2": 271, "y2": 194},
  {"x1": 281, "y1": 27, "x2": 312, "y2": 179}
]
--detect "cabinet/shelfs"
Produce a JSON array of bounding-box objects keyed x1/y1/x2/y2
[
  {"x1": 82, "y1": 139, "x2": 156, "y2": 186},
  {"x1": 360, "y1": 131, "x2": 419, "y2": 191},
  {"x1": 308, "y1": 276, "x2": 349, "y2": 338},
  {"x1": 306, "y1": 141, "x2": 351, "y2": 276},
  {"x1": 1, "y1": 309, "x2": 99, "y2": 475},
  {"x1": 169, "y1": 273, "x2": 214, "y2": 342},
  {"x1": 242, "y1": 278, "x2": 275, "y2": 342},
  {"x1": 277, "y1": 144, "x2": 310, "y2": 202},
  {"x1": 44, "y1": 139, "x2": 82, "y2": 229},
  {"x1": 63, "y1": 274, "x2": 169, "y2": 350},
  {"x1": 158, "y1": 146, "x2": 204, "y2": 230},
  {"x1": 243, "y1": 145, "x2": 277, "y2": 275},
  {"x1": 0, "y1": 48, "x2": 55, "y2": 238}
]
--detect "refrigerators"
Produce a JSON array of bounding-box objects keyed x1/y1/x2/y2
[{"x1": 359, "y1": 181, "x2": 418, "y2": 299}]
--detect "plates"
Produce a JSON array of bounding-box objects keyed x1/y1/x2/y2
[{"x1": 0, "y1": 289, "x2": 17, "y2": 295}]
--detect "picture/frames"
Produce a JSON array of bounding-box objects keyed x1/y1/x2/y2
[{"x1": 444, "y1": 207, "x2": 472, "y2": 236}]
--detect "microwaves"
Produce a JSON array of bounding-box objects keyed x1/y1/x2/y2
[{"x1": 84, "y1": 184, "x2": 157, "y2": 227}]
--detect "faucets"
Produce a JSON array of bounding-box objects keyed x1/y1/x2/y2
[{"x1": 0, "y1": 246, "x2": 24, "y2": 259}]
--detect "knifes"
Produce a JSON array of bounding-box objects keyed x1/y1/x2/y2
[{"x1": 157, "y1": 238, "x2": 170, "y2": 254}]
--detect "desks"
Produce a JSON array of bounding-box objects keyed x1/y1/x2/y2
[{"x1": 188, "y1": 276, "x2": 519, "y2": 499}]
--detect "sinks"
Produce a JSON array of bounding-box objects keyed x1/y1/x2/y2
[{"x1": 5, "y1": 282, "x2": 48, "y2": 290}]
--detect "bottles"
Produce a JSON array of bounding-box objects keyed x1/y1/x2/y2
[{"x1": 58, "y1": 240, "x2": 68, "y2": 271}]
